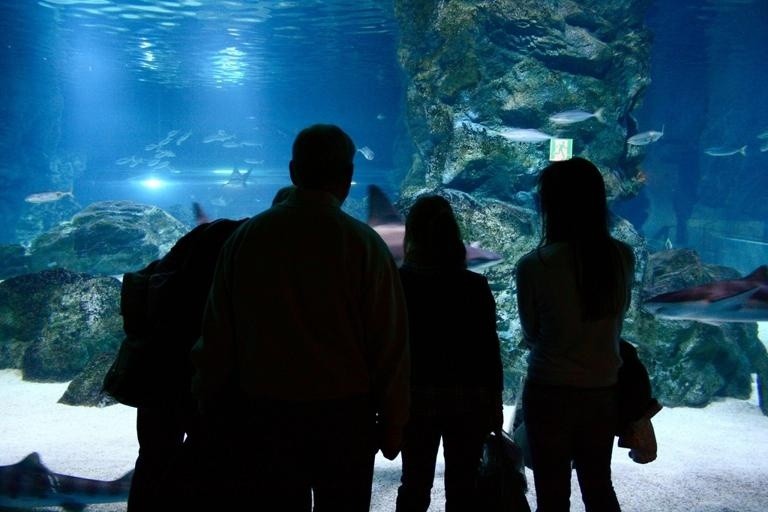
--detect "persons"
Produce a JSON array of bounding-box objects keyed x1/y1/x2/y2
[
  {"x1": 509, "y1": 154, "x2": 638, "y2": 511},
  {"x1": 102, "y1": 185, "x2": 300, "y2": 511},
  {"x1": 381, "y1": 198, "x2": 507, "y2": 511},
  {"x1": 192, "y1": 123, "x2": 405, "y2": 511}
]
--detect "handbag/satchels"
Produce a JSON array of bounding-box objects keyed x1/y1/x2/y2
[
  {"x1": 616, "y1": 339, "x2": 651, "y2": 425},
  {"x1": 444, "y1": 433, "x2": 531, "y2": 512},
  {"x1": 101, "y1": 340, "x2": 147, "y2": 406}
]
